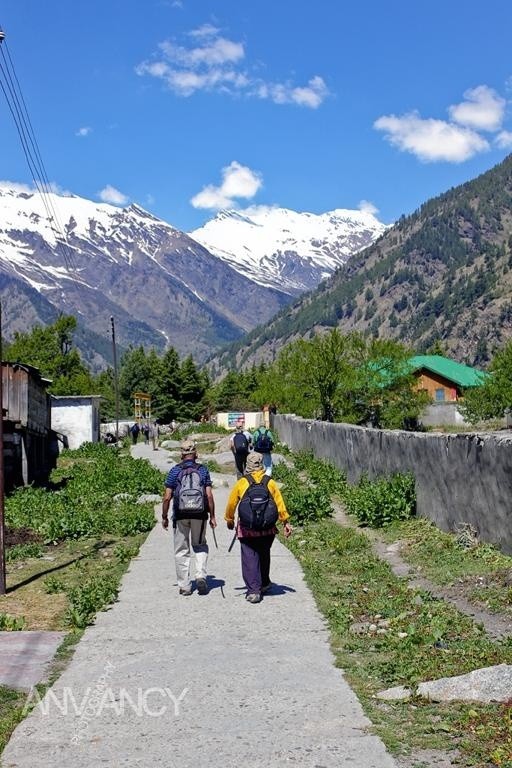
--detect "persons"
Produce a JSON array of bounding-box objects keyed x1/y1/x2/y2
[
  {"x1": 152, "y1": 423, "x2": 159, "y2": 451},
  {"x1": 229, "y1": 420, "x2": 252, "y2": 475},
  {"x1": 223, "y1": 450, "x2": 292, "y2": 603},
  {"x1": 128, "y1": 422, "x2": 141, "y2": 445},
  {"x1": 106, "y1": 433, "x2": 116, "y2": 442},
  {"x1": 249, "y1": 421, "x2": 278, "y2": 478},
  {"x1": 160, "y1": 438, "x2": 218, "y2": 595},
  {"x1": 142, "y1": 423, "x2": 152, "y2": 445}
]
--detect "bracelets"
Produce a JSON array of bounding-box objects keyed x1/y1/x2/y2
[
  {"x1": 283, "y1": 521, "x2": 288, "y2": 528},
  {"x1": 161, "y1": 513, "x2": 168, "y2": 519}
]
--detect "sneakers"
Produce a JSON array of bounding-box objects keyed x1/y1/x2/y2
[
  {"x1": 179, "y1": 589, "x2": 192, "y2": 596},
  {"x1": 260, "y1": 579, "x2": 272, "y2": 592},
  {"x1": 195, "y1": 577, "x2": 209, "y2": 595},
  {"x1": 245, "y1": 593, "x2": 262, "y2": 603}
]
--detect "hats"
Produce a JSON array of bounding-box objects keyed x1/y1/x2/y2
[
  {"x1": 246, "y1": 452, "x2": 264, "y2": 468},
  {"x1": 235, "y1": 423, "x2": 242, "y2": 428},
  {"x1": 259, "y1": 421, "x2": 266, "y2": 427},
  {"x1": 180, "y1": 440, "x2": 196, "y2": 454}
]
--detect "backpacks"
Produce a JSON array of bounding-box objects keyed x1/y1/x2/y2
[
  {"x1": 172, "y1": 462, "x2": 207, "y2": 515},
  {"x1": 254, "y1": 430, "x2": 272, "y2": 452},
  {"x1": 233, "y1": 430, "x2": 247, "y2": 454},
  {"x1": 237, "y1": 473, "x2": 278, "y2": 532}
]
